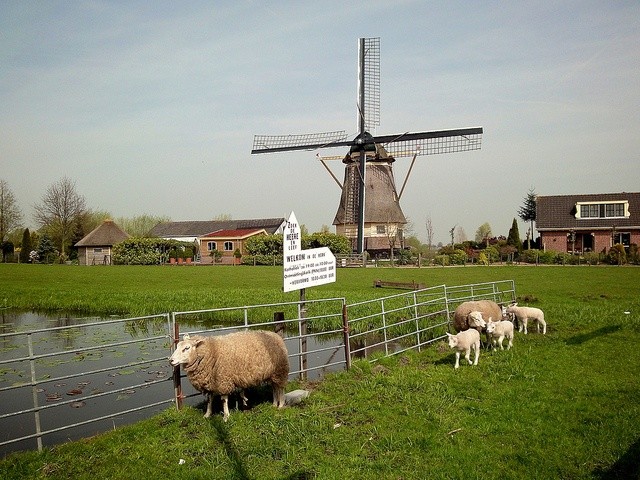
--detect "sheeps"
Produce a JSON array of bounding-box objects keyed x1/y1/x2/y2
[
  {"x1": 446, "y1": 328, "x2": 481, "y2": 368},
  {"x1": 170, "y1": 330, "x2": 289, "y2": 421},
  {"x1": 507, "y1": 303, "x2": 547, "y2": 335},
  {"x1": 454, "y1": 299, "x2": 502, "y2": 349},
  {"x1": 486, "y1": 317, "x2": 514, "y2": 352},
  {"x1": 500, "y1": 305, "x2": 515, "y2": 322}
]
